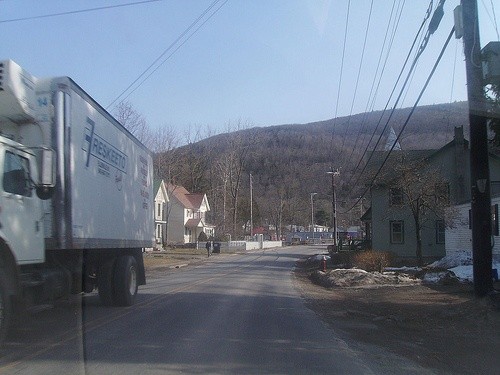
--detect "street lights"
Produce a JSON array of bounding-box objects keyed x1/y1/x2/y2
[{"x1": 311, "y1": 192, "x2": 318, "y2": 244}]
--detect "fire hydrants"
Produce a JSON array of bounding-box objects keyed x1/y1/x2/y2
[{"x1": 321, "y1": 255, "x2": 327, "y2": 272}]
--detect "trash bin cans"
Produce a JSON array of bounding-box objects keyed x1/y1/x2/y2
[{"x1": 213, "y1": 243, "x2": 221, "y2": 253}]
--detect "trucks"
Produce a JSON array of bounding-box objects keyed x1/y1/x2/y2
[{"x1": 0, "y1": 58, "x2": 156, "y2": 350}]
